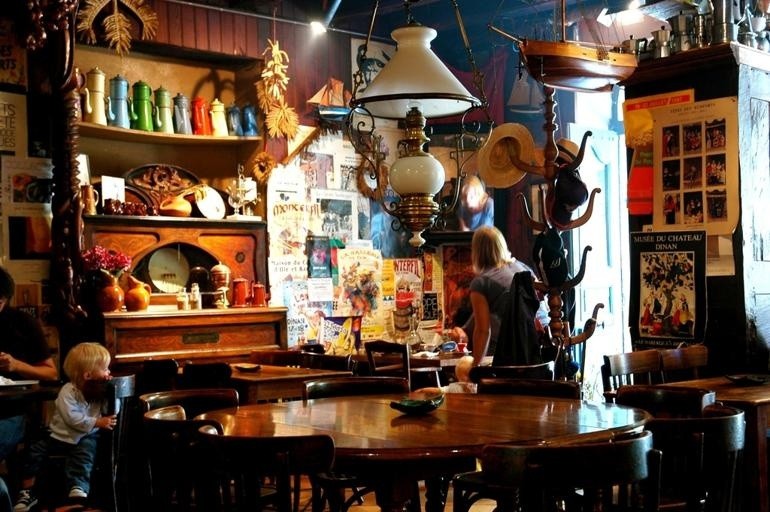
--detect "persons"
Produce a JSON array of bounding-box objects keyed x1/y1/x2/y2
[
  {"x1": 661, "y1": 121, "x2": 727, "y2": 221},
  {"x1": 414, "y1": 355, "x2": 479, "y2": 393},
  {"x1": 12, "y1": 340, "x2": 116, "y2": 512},
  {"x1": 469, "y1": 225, "x2": 557, "y2": 380},
  {"x1": 446, "y1": 270, "x2": 500, "y2": 356},
  {"x1": 0, "y1": 267, "x2": 59, "y2": 451}
]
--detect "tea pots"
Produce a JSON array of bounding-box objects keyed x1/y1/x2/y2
[
  {"x1": 125, "y1": 274, "x2": 153, "y2": 312},
  {"x1": 608, "y1": 1, "x2": 770, "y2": 63},
  {"x1": 96, "y1": 268, "x2": 125, "y2": 312},
  {"x1": 83, "y1": 65, "x2": 259, "y2": 138},
  {"x1": 159, "y1": 191, "x2": 192, "y2": 217}
]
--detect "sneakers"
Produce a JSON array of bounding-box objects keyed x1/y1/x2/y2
[
  {"x1": 69, "y1": 485, "x2": 88, "y2": 497},
  {"x1": 14, "y1": 486, "x2": 39, "y2": 510}
]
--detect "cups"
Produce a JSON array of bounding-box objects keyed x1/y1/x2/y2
[
  {"x1": 81, "y1": 180, "x2": 99, "y2": 216},
  {"x1": 105, "y1": 198, "x2": 158, "y2": 216}
]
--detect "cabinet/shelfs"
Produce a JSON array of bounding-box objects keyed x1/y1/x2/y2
[{"x1": 68, "y1": 38, "x2": 286, "y2": 363}]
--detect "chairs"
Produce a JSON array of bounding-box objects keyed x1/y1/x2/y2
[{"x1": 0, "y1": 334, "x2": 769, "y2": 512}]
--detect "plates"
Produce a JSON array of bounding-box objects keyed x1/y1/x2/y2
[
  {"x1": 724, "y1": 374, "x2": 770, "y2": 386},
  {"x1": 195, "y1": 186, "x2": 227, "y2": 220},
  {"x1": 387, "y1": 392, "x2": 449, "y2": 415},
  {"x1": 148, "y1": 248, "x2": 191, "y2": 293},
  {"x1": 235, "y1": 365, "x2": 261, "y2": 373}
]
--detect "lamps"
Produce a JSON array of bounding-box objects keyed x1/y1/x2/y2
[{"x1": 346, "y1": 0, "x2": 496, "y2": 250}]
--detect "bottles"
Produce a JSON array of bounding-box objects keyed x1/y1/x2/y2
[
  {"x1": 252, "y1": 281, "x2": 268, "y2": 307},
  {"x1": 177, "y1": 285, "x2": 188, "y2": 310},
  {"x1": 232, "y1": 275, "x2": 254, "y2": 308},
  {"x1": 191, "y1": 282, "x2": 202, "y2": 309},
  {"x1": 209, "y1": 261, "x2": 232, "y2": 308},
  {"x1": 457, "y1": 343, "x2": 467, "y2": 352}
]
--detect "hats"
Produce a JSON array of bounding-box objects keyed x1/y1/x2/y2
[
  {"x1": 532, "y1": 223, "x2": 568, "y2": 289},
  {"x1": 477, "y1": 121, "x2": 535, "y2": 190},
  {"x1": 554, "y1": 137, "x2": 580, "y2": 163},
  {"x1": 551, "y1": 171, "x2": 588, "y2": 225}
]
